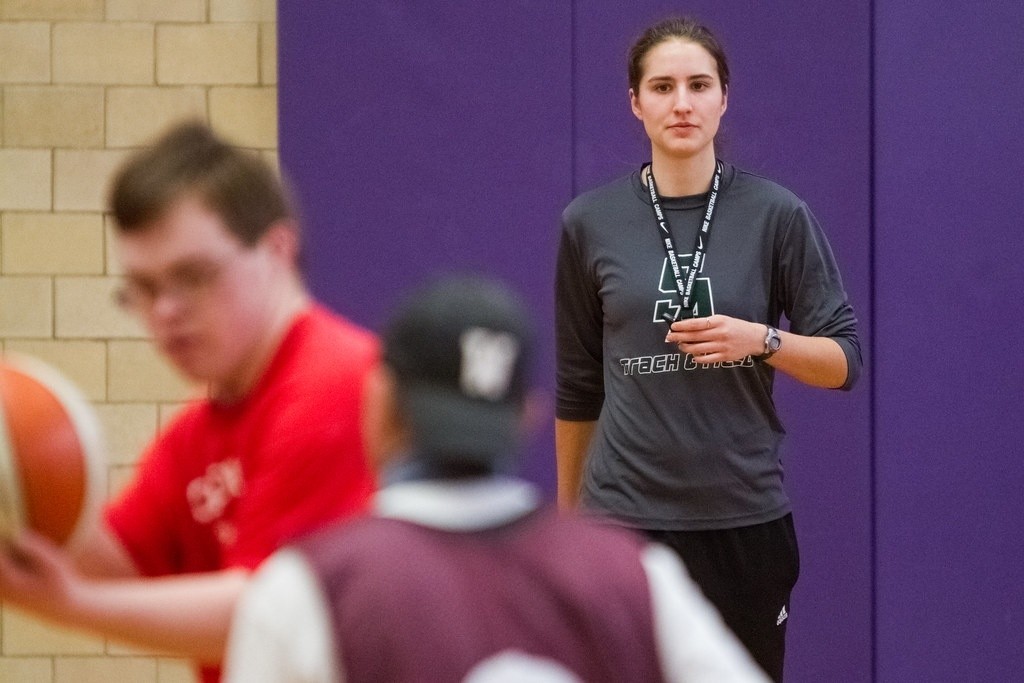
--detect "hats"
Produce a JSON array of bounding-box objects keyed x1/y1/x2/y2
[{"x1": 385, "y1": 277, "x2": 533, "y2": 456}]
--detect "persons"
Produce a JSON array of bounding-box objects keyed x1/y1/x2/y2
[
  {"x1": 552, "y1": 17, "x2": 864, "y2": 683},
  {"x1": 217, "y1": 272, "x2": 779, "y2": 683},
  {"x1": 1, "y1": 115, "x2": 379, "y2": 683}
]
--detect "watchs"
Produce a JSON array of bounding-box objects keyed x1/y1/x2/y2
[{"x1": 750, "y1": 323, "x2": 782, "y2": 362}]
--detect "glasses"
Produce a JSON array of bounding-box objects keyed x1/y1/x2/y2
[{"x1": 107, "y1": 240, "x2": 244, "y2": 321}]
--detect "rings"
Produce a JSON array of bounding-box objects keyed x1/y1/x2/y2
[{"x1": 706, "y1": 317, "x2": 711, "y2": 329}]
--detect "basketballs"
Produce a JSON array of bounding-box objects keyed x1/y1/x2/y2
[{"x1": 0, "y1": 347, "x2": 110, "y2": 582}]
